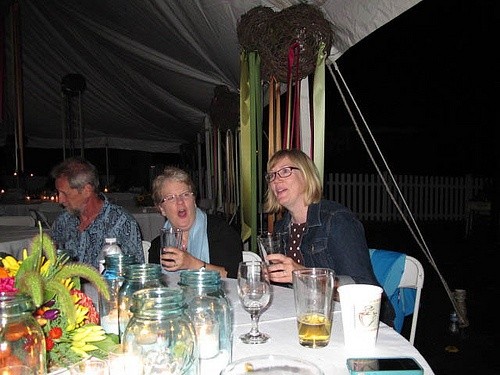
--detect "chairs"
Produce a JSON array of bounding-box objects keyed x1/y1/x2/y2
[
  {"x1": 368, "y1": 249, "x2": 424, "y2": 347},
  {"x1": 28, "y1": 207, "x2": 53, "y2": 230},
  {"x1": 200, "y1": 199, "x2": 237, "y2": 225}
]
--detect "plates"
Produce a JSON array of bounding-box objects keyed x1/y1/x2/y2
[{"x1": 220, "y1": 355, "x2": 323, "y2": 375}]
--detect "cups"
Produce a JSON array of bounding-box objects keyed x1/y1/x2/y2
[
  {"x1": 336, "y1": 284, "x2": 383, "y2": 350},
  {"x1": 292, "y1": 268, "x2": 334, "y2": 348},
  {"x1": 109, "y1": 344, "x2": 143, "y2": 375},
  {"x1": 72, "y1": 360, "x2": 109, "y2": 375},
  {"x1": 160, "y1": 228, "x2": 182, "y2": 268},
  {"x1": 261, "y1": 232, "x2": 286, "y2": 273}
]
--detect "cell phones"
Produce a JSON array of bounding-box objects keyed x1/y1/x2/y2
[{"x1": 346, "y1": 357, "x2": 425, "y2": 375}]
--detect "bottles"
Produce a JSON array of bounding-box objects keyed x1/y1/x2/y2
[
  {"x1": 98, "y1": 238, "x2": 126, "y2": 272},
  {"x1": 177, "y1": 269, "x2": 234, "y2": 375},
  {"x1": 123, "y1": 288, "x2": 200, "y2": 375},
  {"x1": 99, "y1": 254, "x2": 136, "y2": 338},
  {"x1": 118, "y1": 263, "x2": 167, "y2": 345},
  {"x1": 0, "y1": 296, "x2": 47, "y2": 375}
]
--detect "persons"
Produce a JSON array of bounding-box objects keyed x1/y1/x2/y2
[
  {"x1": 263, "y1": 149, "x2": 397, "y2": 331},
  {"x1": 147, "y1": 165, "x2": 248, "y2": 279},
  {"x1": 48, "y1": 155, "x2": 145, "y2": 268}
]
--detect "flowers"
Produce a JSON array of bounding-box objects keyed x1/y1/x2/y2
[{"x1": 0, "y1": 221, "x2": 120, "y2": 372}]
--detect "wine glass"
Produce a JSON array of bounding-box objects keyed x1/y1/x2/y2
[{"x1": 238, "y1": 260, "x2": 273, "y2": 344}]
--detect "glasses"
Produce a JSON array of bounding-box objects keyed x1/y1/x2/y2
[
  {"x1": 265, "y1": 166, "x2": 301, "y2": 183},
  {"x1": 160, "y1": 191, "x2": 194, "y2": 205}
]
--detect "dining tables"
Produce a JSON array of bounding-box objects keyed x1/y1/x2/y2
[
  {"x1": 46, "y1": 278, "x2": 435, "y2": 375},
  {"x1": 0, "y1": 193, "x2": 167, "y2": 264}
]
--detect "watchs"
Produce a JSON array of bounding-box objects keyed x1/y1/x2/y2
[{"x1": 200, "y1": 261, "x2": 206, "y2": 270}]
